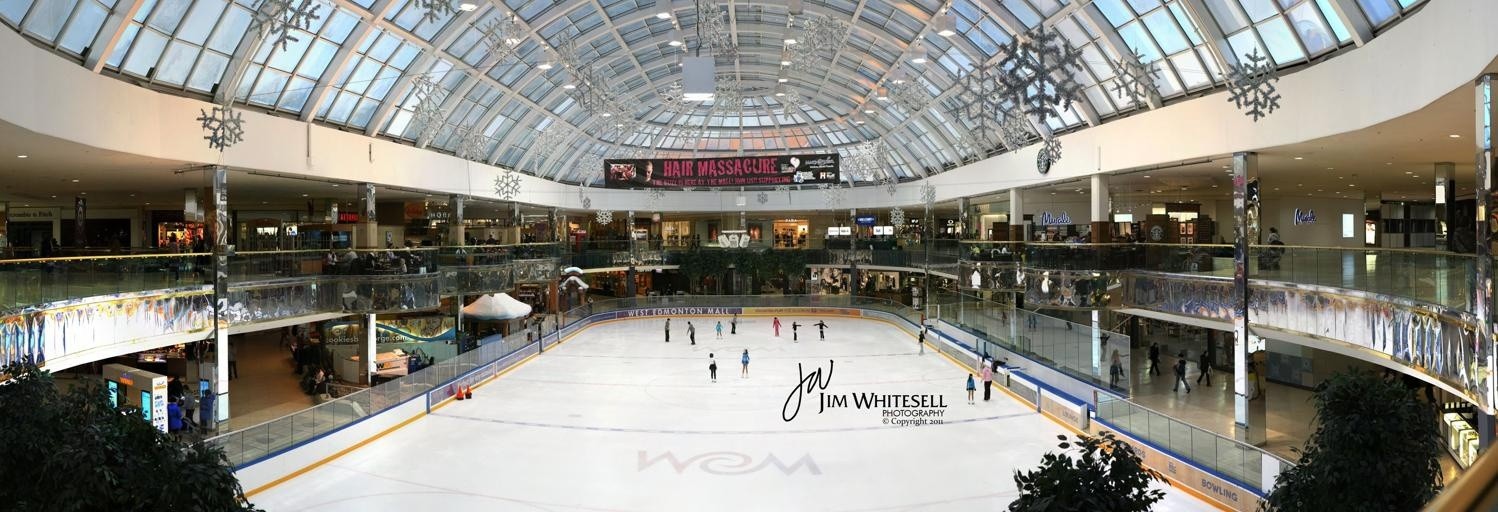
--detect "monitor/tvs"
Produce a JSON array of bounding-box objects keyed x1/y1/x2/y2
[{"x1": 285, "y1": 222, "x2": 297, "y2": 235}]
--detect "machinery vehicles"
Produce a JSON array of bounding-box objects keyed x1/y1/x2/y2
[{"x1": 375, "y1": 347, "x2": 435, "y2": 384}]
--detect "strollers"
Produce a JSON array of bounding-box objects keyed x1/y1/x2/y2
[{"x1": 1257, "y1": 240, "x2": 1285, "y2": 270}]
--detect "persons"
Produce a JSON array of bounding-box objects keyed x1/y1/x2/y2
[
  {"x1": 1027, "y1": 314, "x2": 1032, "y2": 329},
  {"x1": 167, "y1": 373, "x2": 183, "y2": 402},
  {"x1": 228, "y1": 338, "x2": 238, "y2": 381},
  {"x1": 182, "y1": 385, "x2": 197, "y2": 426},
  {"x1": 731, "y1": 313, "x2": 737, "y2": 334},
  {"x1": 792, "y1": 321, "x2": 805, "y2": 343},
  {"x1": 918, "y1": 330, "x2": 926, "y2": 355},
  {"x1": 1032, "y1": 314, "x2": 1037, "y2": 328},
  {"x1": 628, "y1": 160, "x2": 656, "y2": 190},
  {"x1": 1000, "y1": 311, "x2": 1008, "y2": 328},
  {"x1": 979, "y1": 358, "x2": 994, "y2": 402},
  {"x1": 685, "y1": 321, "x2": 696, "y2": 345},
  {"x1": 813, "y1": 319, "x2": 829, "y2": 341},
  {"x1": 304, "y1": 367, "x2": 325, "y2": 396},
  {"x1": 1100, "y1": 331, "x2": 1112, "y2": 362},
  {"x1": 1171, "y1": 350, "x2": 1192, "y2": 394},
  {"x1": 1065, "y1": 321, "x2": 1074, "y2": 329},
  {"x1": 1109, "y1": 347, "x2": 1125, "y2": 390},
  {"x1": 965, "y1": 373, "x2": 977, "y2": 406},
  {"x1": 741, "y1": 348, "x2": 750, "y2": 378},
  {"x1": 325, "y1": 233, "x2": 508, "y2": 274},
  {"x1": 1147, "y1": 340, "x2": 1161, "y2": 377},
  {"x1": 716, "y1": 320, "x2": 723, "y2": 336},
  {"x1": 199, "y1": 388, "x2": 216, "y2": 430},
  {"x1": 708, "y1": 352, "x2": 718, "y2": 383},
  {"x1": 772, "y1": 317, "x2": 782, "y2": 337},
  {"x1": 587, "y1": 296, "x2": 594, "y2": 314},
  {"x1": 664, "y1": 317, "x2": 671, "y2": 342},
  {"x1": 166, "y1": 396, "x2": 184, "y2": 445},
  {"x1": 1196, "y1": 349, "x2": 1213, "y2": 388},
  {"x1": 165, "y1": 233, "x2": 181, "y2": 282},
  {"x1": 1265, "y1": 226, "x2": 1286, "y2": 270}
]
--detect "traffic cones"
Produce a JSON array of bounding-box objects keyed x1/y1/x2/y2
[
  {"x1": 465, "y1": 385, "x2": 472, "y2": 399},
  {"x1": 447, "y1": 383, "x2": 454, "y2": 395},
  {"x1": 456, "y1": 385, "x2": 464, "y2": 401}
]
